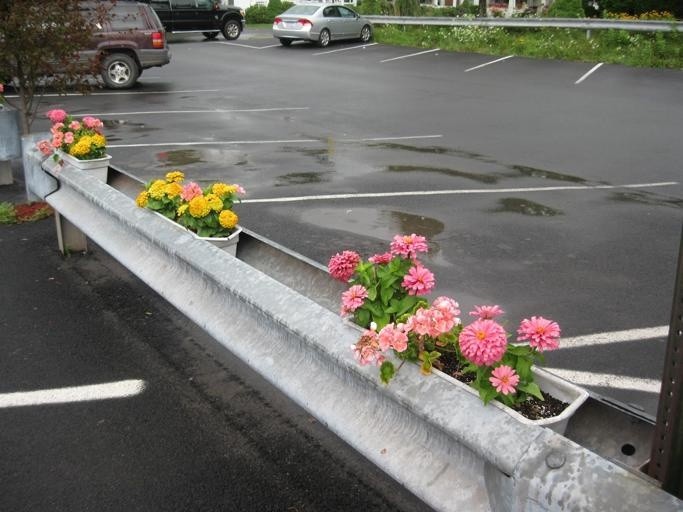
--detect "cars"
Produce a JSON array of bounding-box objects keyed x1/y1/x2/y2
[{"x1": 272, "y1": 2, "x2": 374, "y2": 48}]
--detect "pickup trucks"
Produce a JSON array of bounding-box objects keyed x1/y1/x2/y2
[{"x1": 149, "y1": 0, "x2": 248, "y2": 46}]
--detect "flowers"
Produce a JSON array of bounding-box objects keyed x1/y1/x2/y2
[
  {"x1": 135, "y1": 170, "x2": 246, "y2": 239},
  {"x1": 36, "y1": 109, "x2": 108, "y2": 156},
  {"x1": 328, "y1": 231, "x2": 563, "y2": 408}
]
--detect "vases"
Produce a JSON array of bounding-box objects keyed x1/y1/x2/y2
[
  {"x1": 143, "y1": 206, "x2": 243, "y2": 258},
  {"x1": 341, "y1": 314, "x2": 590, "y2": 436},
  {"x1": 55, "y1": 149, "x2": 113, "y2": 184}
]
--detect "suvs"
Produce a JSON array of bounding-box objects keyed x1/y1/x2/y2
[{"x1": 0, "y1": 0, "x2": 172, "y2": 93}]
